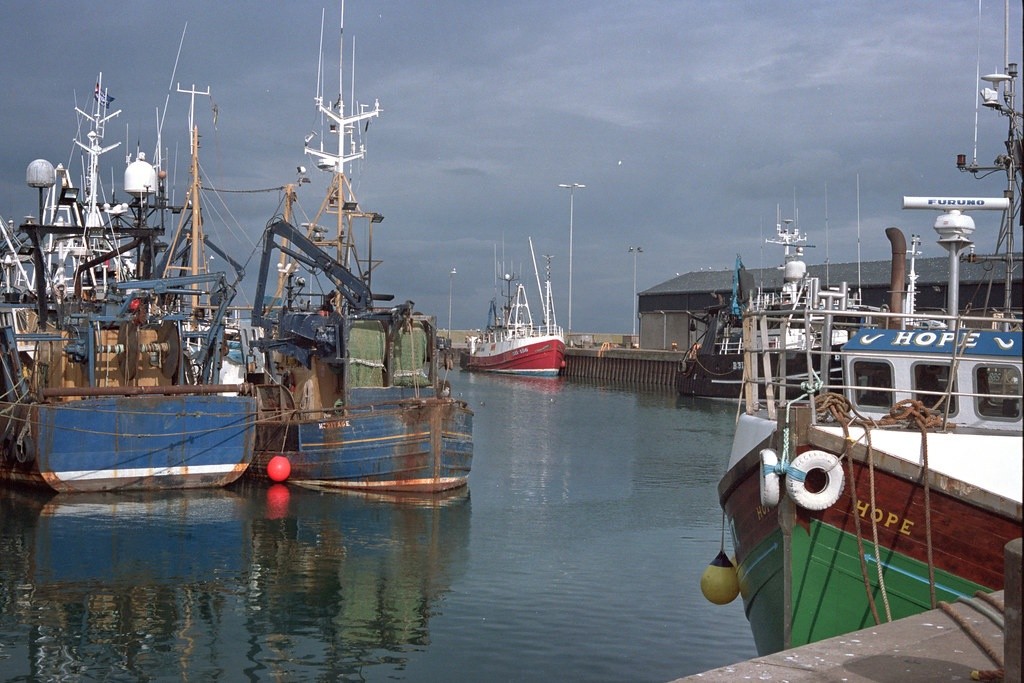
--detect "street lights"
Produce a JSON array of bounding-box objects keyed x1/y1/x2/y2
[
  {"x1": 685, "y1": 310, "x2": 700, "y2": 341},
  {"x1": 557, "y1": 183, "x2": 585, "y2": 334},
  {"x1": 447, "y1": 268, "x2": 457, "y2": 338},
  {"x1": 628, "y1": 246, "x2": 644, "y2": 333},
  {"x1": 656, "y1": 311, "x2": 667, "y2": 348}
]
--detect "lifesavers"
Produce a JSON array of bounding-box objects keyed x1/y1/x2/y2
[
  {"x1": 754, "y1": 449, "x2": 785, "y2": 511},
  {"x1": 784, "y1": 448, "x2": 845, "y2": 512}
]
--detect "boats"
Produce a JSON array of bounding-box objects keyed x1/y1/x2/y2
[
  {"x1": 702, "y1": 0, "x2": 1023, "y2": 655},
  {"x1": 0, "y1": 21, "x2": 266, "y2": 491},
  {"x1": 239, "y1": 0, "x2": 474, "y2": 507},
  {"x1": 460, "y1": 231, "x2": 566, "y2": 378}
]
